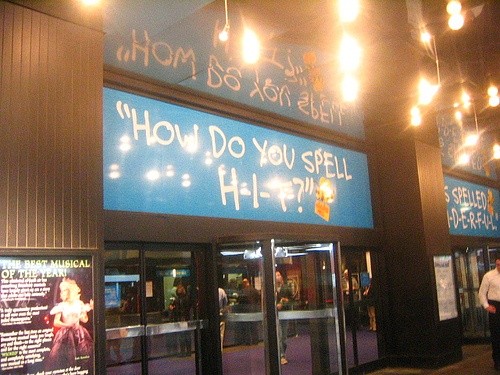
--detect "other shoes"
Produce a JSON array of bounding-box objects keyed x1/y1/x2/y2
[{"x1": 281, "y1": 358, "x2": 288, "y2": 365}]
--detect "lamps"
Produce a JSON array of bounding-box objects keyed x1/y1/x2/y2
[{"x1": 218, "y1": 0, "x2": 231, "y2": 42}]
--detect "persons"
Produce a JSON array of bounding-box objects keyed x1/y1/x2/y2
[
  {"x1": 275, "y1": 271, "x2": 294, "y2": 364},
  {"x1": 344, "y1": 268, "x2": 376, "y2": 331},
  {"x1": 236, "y1": 278, "x2": 259, "y2": 346},
  {"x1": 168, "y1": 280, "x2": 192, "y2": 357},
  {"x1": 45, "y1": 277, "x2": 94, "y2": 374},
  {"x1": 478, "y1": 250, "x2": 500, "y2": 371},
  {"x1": 218, "y1": 288, "x2": 228, "y2": 353}
]
